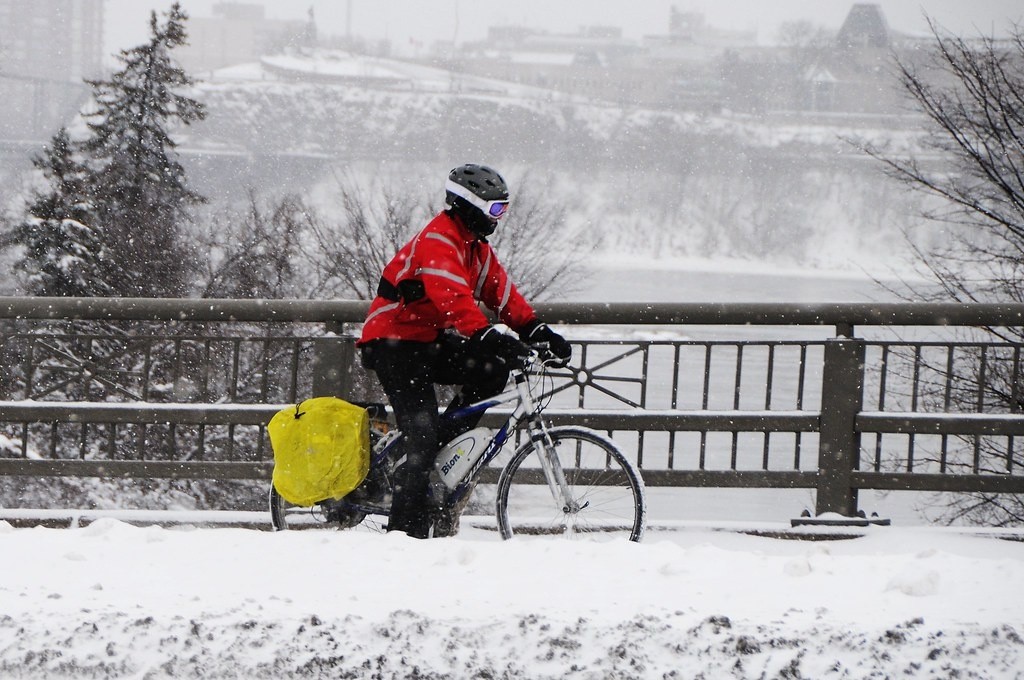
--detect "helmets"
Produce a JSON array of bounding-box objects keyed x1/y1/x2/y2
[{"x1": 445, "y1": 164, "x2": 509, "y2": 236}]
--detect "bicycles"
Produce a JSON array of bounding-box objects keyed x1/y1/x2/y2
[{"x1": 266, "y1": 340, "x2": 646, "y2": 544}]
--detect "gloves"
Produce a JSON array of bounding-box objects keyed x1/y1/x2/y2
[
  {"x1": 519, "y1": 319, "x2": 572, "y2": 368},
  {"x1": 462, "y1": 323, "x2": 529, "y2": 370}
]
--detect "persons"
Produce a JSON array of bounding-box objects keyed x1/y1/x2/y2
[{"x1": 355, "y1": 165, "x2": 571, "y2": 538}]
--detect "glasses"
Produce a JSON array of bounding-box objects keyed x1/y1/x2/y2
[{"x1": 484, "y1": 199, "x2": 510, "y2": 219}]
